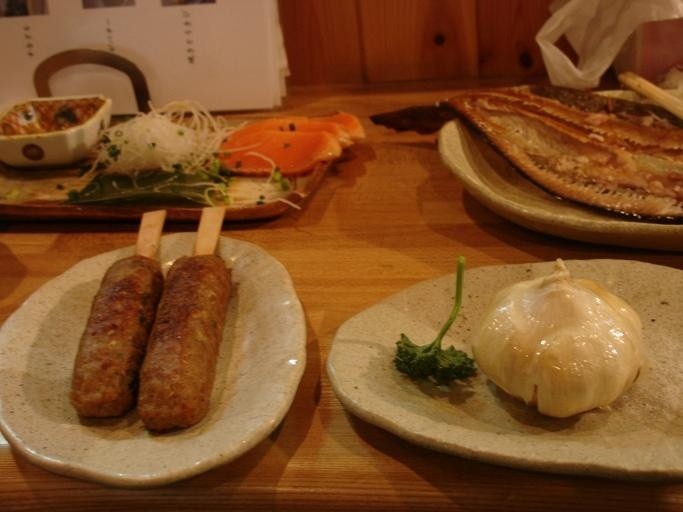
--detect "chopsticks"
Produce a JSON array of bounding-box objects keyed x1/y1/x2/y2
[{"x1": 618, "y1": 69, "x2": 683, "y2": 124}]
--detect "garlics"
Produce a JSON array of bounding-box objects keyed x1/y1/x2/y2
[{"x1": 471, "y1": 258, "x2": 645, "y2": 420}]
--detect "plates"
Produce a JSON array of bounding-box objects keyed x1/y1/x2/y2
[
  {"x1": 328, "y1": 260, "x2": 682, "y2": 488},
  {"x1": 436, "y1": 89, "x2": 680, "y2": 251},
  {"x1": 4, "y1": 232, "x2": 306, "y2": 488},
  {"x1": 1, "y1": 116, "x2": 344, "y2": 220}
]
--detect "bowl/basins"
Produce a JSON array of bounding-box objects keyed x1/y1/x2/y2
[{"x1": 0, "y1": 94, "x2": 111, "y2": 167}]
